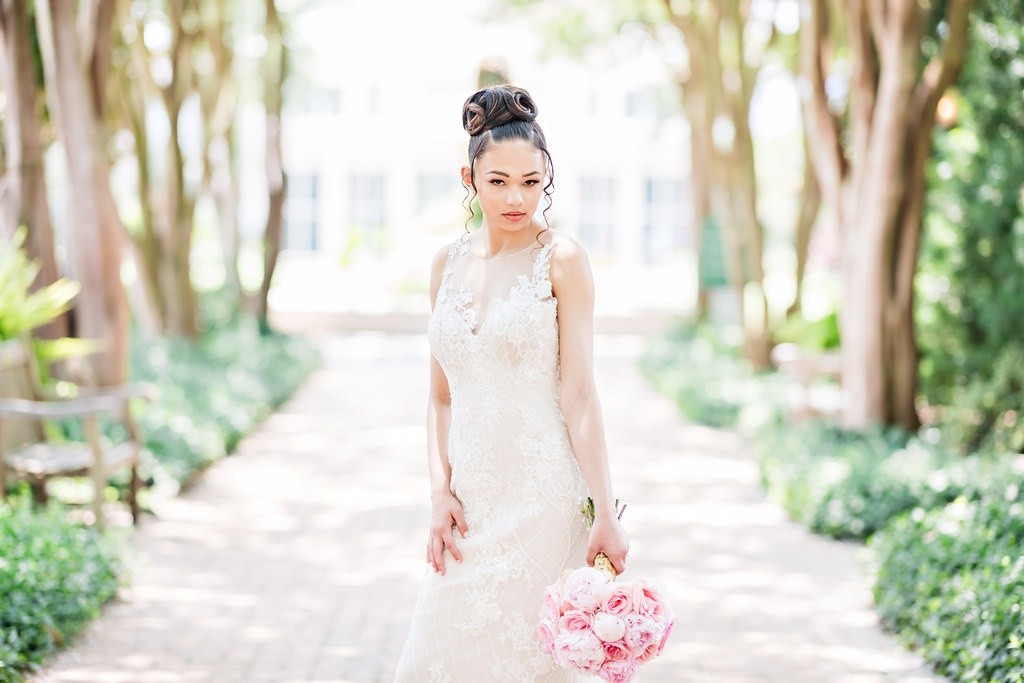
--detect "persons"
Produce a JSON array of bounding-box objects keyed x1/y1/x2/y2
[{"x1": 395, "y1": 85, "x2": 630, "y2": 683}]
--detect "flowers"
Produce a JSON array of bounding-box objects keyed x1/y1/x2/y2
[{"x1": 538, "y1": 496, "x2": 675, "y2": 683}]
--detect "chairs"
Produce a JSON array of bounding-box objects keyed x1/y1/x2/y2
[{"x1": 0, "y1": 332, "x2": 142, "y2": 530}]
